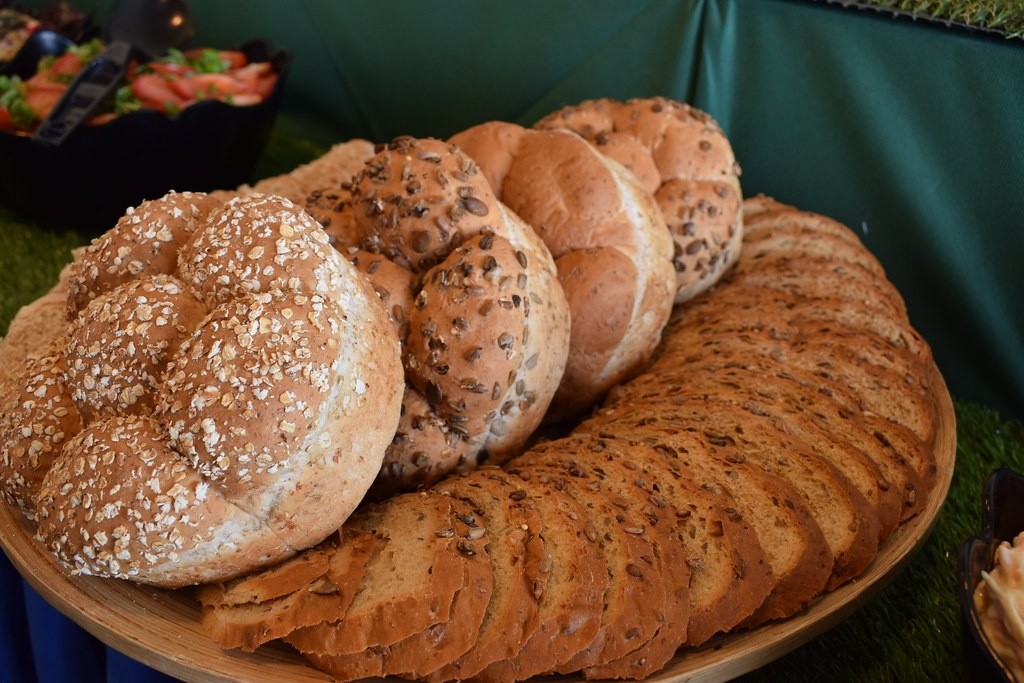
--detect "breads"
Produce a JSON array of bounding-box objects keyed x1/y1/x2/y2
[{"x1": 0, "y1": 96, "x2": 941, "y2": 683}]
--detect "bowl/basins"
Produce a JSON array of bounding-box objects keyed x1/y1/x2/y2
[{"x1": 0, "y1": 38, "x2": 293, "y2": 232}]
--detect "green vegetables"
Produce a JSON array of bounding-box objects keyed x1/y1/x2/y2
[{"x1": 0, "y1": 37, "x2": 234, "y2": 126}]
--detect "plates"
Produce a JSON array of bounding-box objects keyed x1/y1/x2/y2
[
  {"x1": 0, "y1": 361, "x2": 955, "y2": 683},
  {"x1": 959, "y1": 464, "x2": 1024, "y2": 683}
]
